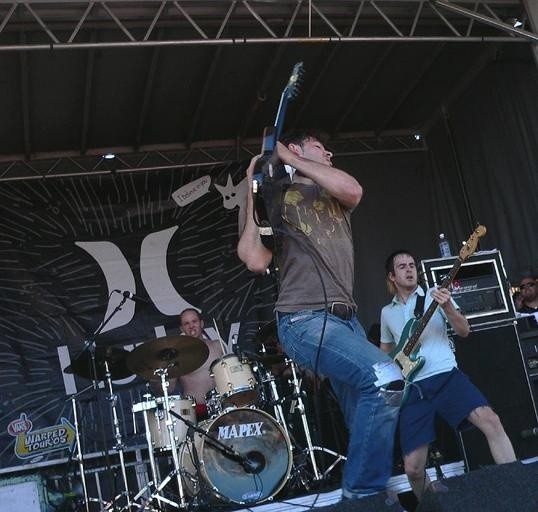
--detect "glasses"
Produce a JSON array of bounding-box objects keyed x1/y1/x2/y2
[{"x1": 520, "y1": 282, "x2": 537, "y2": 290}]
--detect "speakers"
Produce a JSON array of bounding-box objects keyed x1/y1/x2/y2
[
  {"x1": 448, "y1": 323, "x2": 538, "y2": 472},
  {"x1": 414, "y1": 458, "x2": 538, "y2": 512}
]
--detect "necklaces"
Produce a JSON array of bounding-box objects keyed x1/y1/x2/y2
[{"x1": 527, "y1": 305, "x2": 537, "y2": 310}]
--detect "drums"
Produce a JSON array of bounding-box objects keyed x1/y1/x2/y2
[
  {"x1": 209, "y1": 353, "x2": 260, "y2": 407},
  {"x1": 147, "y1": 395, "x2": 196, "y2": 452},
  {"x1": 178, "y1": 407, "x2": 293, "y2": 508}
]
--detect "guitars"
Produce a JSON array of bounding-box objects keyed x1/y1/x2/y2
[
  {"x1": 390, "y1": 222, "x2": 486, "y2": 405},
  {"x1": 253, "y1": 62, "x2": 306, "y2": 228}
]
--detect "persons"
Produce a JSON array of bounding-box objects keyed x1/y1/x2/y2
[
  {"x1": 372, "y1": 247, "x2": 517, "y2": 500},
  {"x1": 151, "y1": 306, "x2": 232, "y2": 506},
  {"x1": 235, "y1": 128, "x2": 405, "y2": 512},
  {"x1": 513, "y1": 276, "x2": 537, "y2": 315}
]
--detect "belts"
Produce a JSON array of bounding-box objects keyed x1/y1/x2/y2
[{"x1": 327, "y1": 302, "x2": 358, "y2": 321}]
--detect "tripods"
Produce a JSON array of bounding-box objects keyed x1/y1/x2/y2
[{"x1": 76, "y1": 361, "x2": 347, "y2": 512}]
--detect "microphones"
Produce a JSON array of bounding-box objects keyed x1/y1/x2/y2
[{"x1": 123, "y1": 291, "x2": 149, "y2": 304}]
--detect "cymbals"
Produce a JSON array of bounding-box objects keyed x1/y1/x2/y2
[
  {"x1": 126, "y1": 336, "x2": 209, "y2": 383},
  {"x1": 252, "y1": 320, "x2": 289, "y2": 363},
  {"x1": 71, "y1": 346, "x2": 135, "y2": 380}
]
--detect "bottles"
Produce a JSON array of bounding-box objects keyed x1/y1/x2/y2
[{"x1": 438, "y1": 234, "x2": 452, "y2": 259}]
